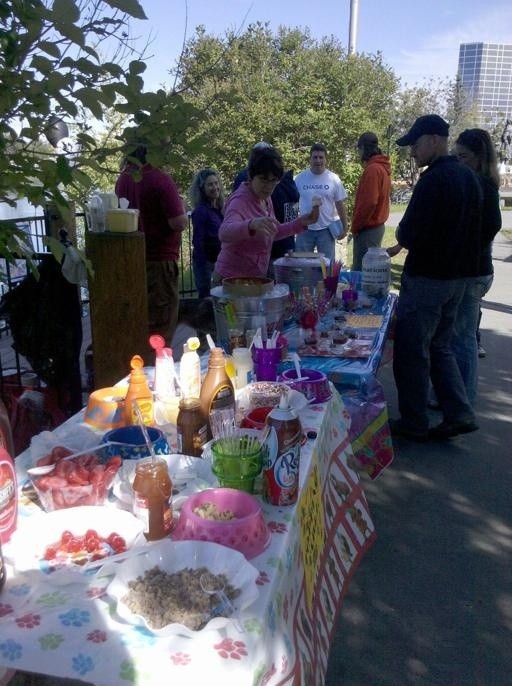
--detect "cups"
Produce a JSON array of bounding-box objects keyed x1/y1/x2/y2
[
  {"x1": 249, "y1": 341, "x2": 287, "y2": 379},
  {"x1": 343, "y1": 290, "x2": 358, "y2": 306},
  {"x1": 297, "y1": 317, "x2": 362, "y2": 352},
  {"x1": 105, "y1": 207, "x2": 137, "y2": 235}
]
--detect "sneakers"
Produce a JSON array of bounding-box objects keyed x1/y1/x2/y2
[
  {"x1": 477, "y1": 342, "x2": 487, "y2": 359},
  {"x1": 388, "y1": 417, "x2": 429, "y2": 444},
  {"x1": 428, "y1": 410, "x2": 482, "y2": 441}
]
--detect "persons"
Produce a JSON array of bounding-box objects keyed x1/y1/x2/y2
[
  {"x1": 212, "y1": 148, "x2": 319, "y2": 289},
  {"x1": 234, "y1": 142, "x2": 300, "y2": 258},
  {"x1": 350, "y1": 133, "x2": 391, "y2": 270},
  {"x1": 426, "y1": 128, "x2": 501, "y2": 409},
  {"x1": 474, "y1": 310, "x2": 486, "y2": 356},
  {"x1": 114, "y1": 143, "x2": 188, "y2": 358},
  {"x1": 388, "y1": 114, "x2": 481, "y2": 441},
  {"x1": 292, "y1": 143, "x2": 348, "y2": 262},
  {"x1": 191, "y1": 169, "x2": 226, "y2": 296}
]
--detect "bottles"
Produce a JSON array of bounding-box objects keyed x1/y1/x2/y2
[
  {"x1": 263, "y1": 393, "x2": 302, "y2": 508},
  {"x1": 358, "y1": 246, "x2": 392, "y2": 298},
  {"x1": 84, "y1": 193, "x2": 107, "y2": 234},
  {"x1": 126, "y1": 336, "x2": 238, "y2": 459}
]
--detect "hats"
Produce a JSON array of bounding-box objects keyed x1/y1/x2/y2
[
  {"x1": 396, "y1": 114, "x2": 452, "y2": 147},
  {"x1": 357, "y1": 132, "x2": 379, "y2": 148}
]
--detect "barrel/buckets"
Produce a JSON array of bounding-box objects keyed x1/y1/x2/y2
[
  {"x1": 362, "y1": 247, "x2": 391, "y2": 297},
  {"x1": 210, "y1": 283, "x2": 289, "y2": 343},
  {"x1": 273, "y1": 252, "x2": 331, "y2": 295}
]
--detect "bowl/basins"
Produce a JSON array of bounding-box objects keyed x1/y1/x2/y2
[
  {"x1": 241, "y1": 407, "x2": 308, "y2": 446},
  {"x1": 94, "y1": 425, "x2": 171, "y2": 460},
  {"x1": 247, "y1": 381, "x2": 290, "y2": 406},
  {"x1": 171, "y1": 488, "x2": 272, "y2": 563},
  {"x1": 276, "y1": 366, "x2": 333, "y2": 404},
  {"x1": 82, "y1": 388, "x2": 128, "y2": 431}
]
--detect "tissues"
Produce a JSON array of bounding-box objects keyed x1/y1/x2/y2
[{"x1": 107, "y1": 198, "x2": 139, "y2": 232}]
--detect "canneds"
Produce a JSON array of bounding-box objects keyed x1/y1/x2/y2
[
  {"x1": 177, "y1": 397, "x2": 208, "y2": 457},
  {"x1": 131, "y1": 459, "x2": 174, "y2": 541}
]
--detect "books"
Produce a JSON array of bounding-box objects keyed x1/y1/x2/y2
[{"x1": 328, "y1": 220, "x2": 343, "y2": 238}]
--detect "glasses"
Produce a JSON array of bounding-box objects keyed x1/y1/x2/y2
[{"x1": 256, "y1": 173, "x2": 280, "y2": 185}]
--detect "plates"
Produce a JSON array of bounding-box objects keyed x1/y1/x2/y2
[
  {"x1": 13, "y1": 505, "x2": 148, "y2": 603},
  {"x1": 114, "y1": 537, "x2": 260, "y2": 633}
]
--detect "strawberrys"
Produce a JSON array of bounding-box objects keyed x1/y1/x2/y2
[{"x1": 33, "y1": 446, "x2": 122, "y2": 507}]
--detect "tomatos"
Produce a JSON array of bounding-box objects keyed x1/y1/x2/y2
[{"x1": 44, "y1": 529, "x2": 127, "y2": 566}]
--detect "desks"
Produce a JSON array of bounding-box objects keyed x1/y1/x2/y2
[
  {"x1": 270, "y1": 291, "x2": 400, "y2": 478},
  {"x1": 1, "y1": 360, "x2": 380, "y2": 685}
]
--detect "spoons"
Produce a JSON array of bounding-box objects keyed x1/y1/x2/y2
[{"x1": 25, "y1": 441, "x2": 115, "y2": 476}]
